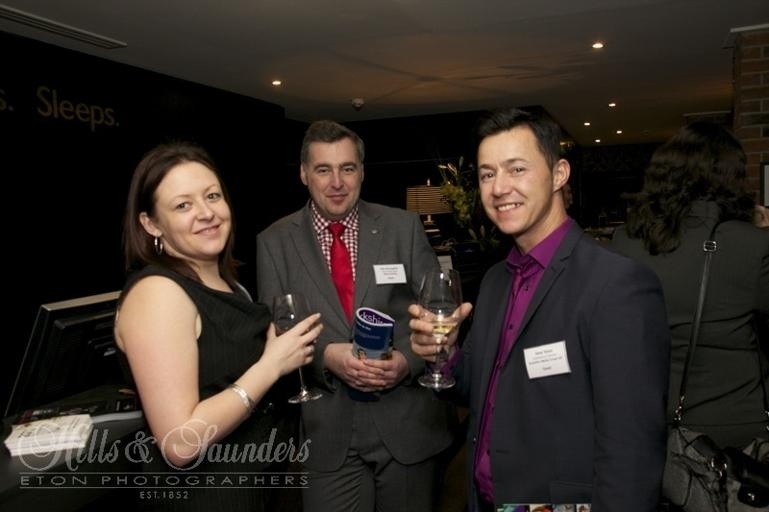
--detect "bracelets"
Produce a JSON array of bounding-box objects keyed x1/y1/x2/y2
[{"x1": 227, "y1": 383, "x2": 256, "y2": 416}]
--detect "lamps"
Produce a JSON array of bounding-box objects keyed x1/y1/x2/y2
[{"x1": 405, "y1": 186, "x2": 455, "y2": 242}]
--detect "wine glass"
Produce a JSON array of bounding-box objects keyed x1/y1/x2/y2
[
  {"x1": 272, "y1": 292, "x2": 323, "y2": 404},
  {"x1": 417, "y1": 268, "x2": 463, "y2": 390}
]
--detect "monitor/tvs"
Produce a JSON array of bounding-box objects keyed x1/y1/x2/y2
[{"x1": 4, "y1": 289, "x2": 121, "y2": 419}]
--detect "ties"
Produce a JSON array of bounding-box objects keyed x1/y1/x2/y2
[{"x1": 328, "y1": 223, "x2": 356, "y2": 326}]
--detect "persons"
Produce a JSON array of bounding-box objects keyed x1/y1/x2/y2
[
  {"x1": 610, "y1": 116, "x2": 769, "y2": 512},
  {"x1": 407, "y1": 100, "x2": 672, "y2": 512},
  {"x1": 109, "y1": 143, "x2": 328, "y2": 511},
  {"x1": 254, "y1": 120, "x2": 456, "y2": 511}
]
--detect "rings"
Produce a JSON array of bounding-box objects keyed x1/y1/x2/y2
[{"x1": 307, "y1": 345, "x2": 312, "y2": 354}]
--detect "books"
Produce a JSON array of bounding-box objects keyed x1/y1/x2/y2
[{"x1": 347, "y1": 306, "x2": 396, "y2": 403}]
